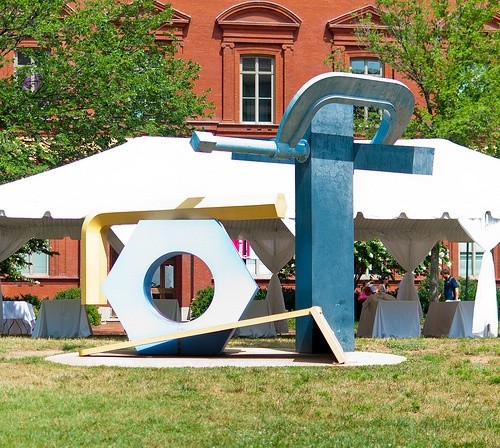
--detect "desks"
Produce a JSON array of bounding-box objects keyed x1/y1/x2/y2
[
  {"x1": 3, "y1": 301, "x2": 30, "y2": 336},
  {"x1": 154, "y1": 298, "x2": 179, "y2": 321},
  {"x1": 236, "y1": 300, "x2": 272, "y2": 336},
  {"x1": 42, "y1": 299, "x2": 83, "y2": 338},
  {"x1": 362, "y1": 300, "x2": 420, "y2": 340},
  {"x1": 431, "y1": 302, "x2": 487, "y2": 337}
]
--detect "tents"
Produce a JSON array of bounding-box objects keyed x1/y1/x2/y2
[{"x1": 0, "y1": 135, "x2": 500, "y2": 341}]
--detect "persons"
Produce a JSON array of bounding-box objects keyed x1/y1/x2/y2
[
  {"x1": 377, "y1": 277, "x2": 389, "y2": 292},
  {"x1": 438, "y1": 269, "x2": 460, "y2": 301}
]
